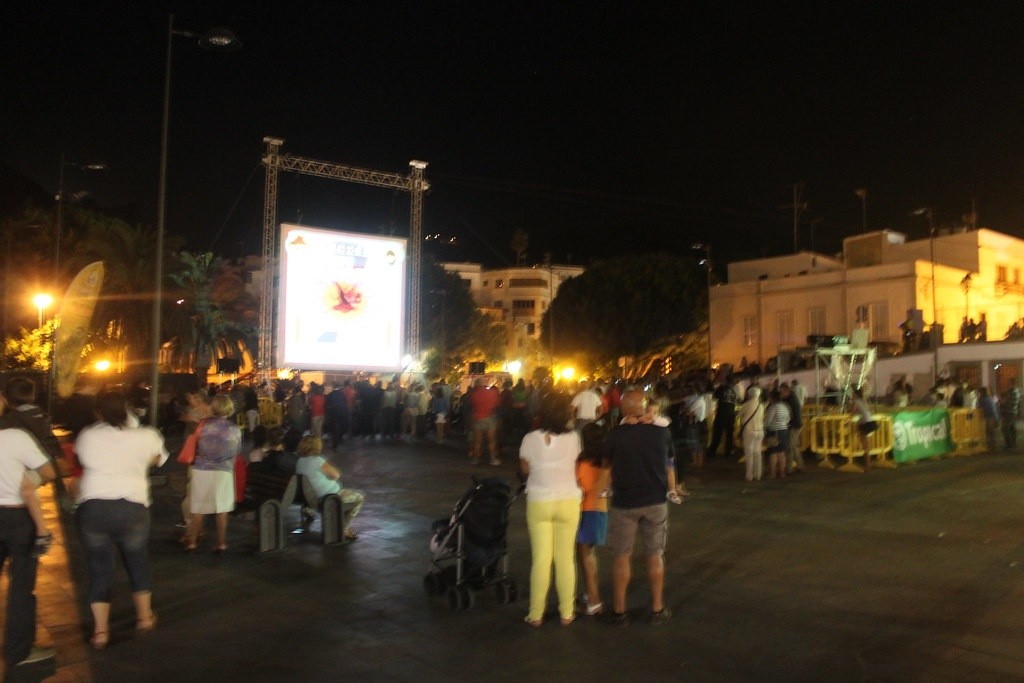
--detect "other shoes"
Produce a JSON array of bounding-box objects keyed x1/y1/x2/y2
[
  {"x1": 468, "y1": 450, "x2": 481, "y2": 457},
  {"x1": 89, "y1": 633, "x2": 110, "y2": 651},
  {"x1": 597, "y1": 488, "x2": 614, "y2": 499},
  {"x1": 490, "y1": 458, "x2": 502, "y2": 466},
  {"x1": 524, "y1": 615, "x2": 542, "y2": 629},
  {"x1": 651, "y1": 608, "x2": 672, "y2": 626},
  {"x1": 584, "y1": 602, "x2": 603, "y2": 616},
  {"x1": 30, "y1": 534, "x2": 53, "y2": 558},
  {"x1": 17, "y1": 645, "x2": 58, "y2": 667},
  {"x1": 613, "y1": 613, "x2": 629, "y2": 627},
  {"x1": 667, "y1": 490, "x2": 683, "y2": 506},
  {"x1": 345, "y1": 530, "x2": 358, "y2": 539},
  {"x1": 184, "y1": 543, "x2": 197, "y2": 552},
  {"x1": 560, "y1": 617, "x2": 574, "y2": 627},
  {"x1": 212, "y1": 542, "x2": 226, "y2": 554},
  {"x1": 136, "y1": 613, "x2": 158, "y2": 631}
]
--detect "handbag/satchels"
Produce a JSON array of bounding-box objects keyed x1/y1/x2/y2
[
  {"x1": 860, "y1": 421, "x2": 878, "y2": 434},
  {"x1": 234, "y1": 454, "x2": 247, "y2": 503},
  {"x1": 737, "y1": 428, "x2": 743, "y2": 439},
  {"x1": 178, "y1": 419, "x2": 204, "y2": 465}
]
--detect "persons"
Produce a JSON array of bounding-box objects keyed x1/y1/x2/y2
[
  {"x1": 956, "y1": 311, "x2": 987, "y2": 345},
  {"x1": 920, "y1": 374, "x2": 997, "y2": 454},
  {"x1": 566, "y1": 374, "x2": 660, "y2": 427},
  {"x1": 1, "y1": 377, "x2": 65, "y2": 668},
  {"x1": 70, "y1": 386, "x2": 169, "y2": 651},
  {"x1": 519, "y1": 383, "x2": 684, "y2": 627},
  {"x1": 848, "y1": 389, "x2": 878, "y2": 465},
  {"x1": 454, "y1": 365, "x2": 565, "y2": 466},
  {"x1": 352, "y1": 379, "x2": 454, "y2": 440},
  {"x1": 890, "y1": 380, "x2": 914, "y2": 408},
  {"x1": 170, "y1": 381, "x2": 366, "y2": 550},
  {"x1": 997, "y1": 376, "x2": 1019, "y2": 449},
  {"x1": 648, "y1": 364, "x2": 807, "y2": 491}
]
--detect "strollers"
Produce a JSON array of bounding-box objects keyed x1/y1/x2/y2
[{"x1": 429, "y1": 472, "x2": 526, "y2": 614}]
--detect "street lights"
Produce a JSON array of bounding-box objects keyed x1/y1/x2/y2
[
  {"x1": 33, "y1": 292, "x2": 52, "y2": 330},
  {"x1": 534, "y1": 263, "x2": 553, "y2": 383},
  {"x1": 150, "y1": 19, "x2": 231, "y2": 429},
  {"x1": 45, "y1": 157, "x2": 105, "y2": 436},
  {"x1": 693, "y1": 240, "x2": 711, "y2": 380},
  {"x1": 428, "y1": 288, "x2": 445, "y2": 377},
  {"x1": 910, "y1": 206, "x2": 937, "y2": 382}
]
